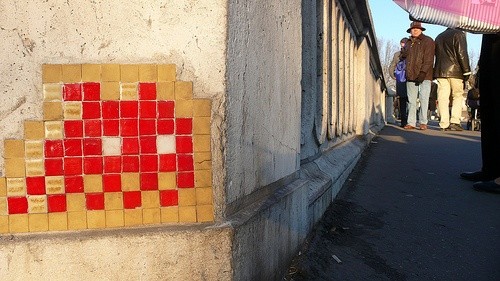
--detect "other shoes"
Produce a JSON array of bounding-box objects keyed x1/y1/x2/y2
[
  {"x1": 460, "y1": 169, "x2": 500, "y2": 193},
  {"x1": 420, "y1": 124, "x2": 428, "y2": 130},
  {"x1": 404, "y1": 126, "x2": 415, "y2": 129},
  {"x1": 441, "y1": 123, "x2": 463, "y2": 131}
]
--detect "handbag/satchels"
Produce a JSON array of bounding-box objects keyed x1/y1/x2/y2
[{"x1": 393, "y1": 59, "x2": 406, "y2": 83}]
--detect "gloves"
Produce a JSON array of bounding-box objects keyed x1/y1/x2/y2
[
  {"x1": 417, "y1": 72, "x2": 425, "y2": 83},
  {"x1": 464, "y1": 75, "x2": 470, "y2": 81}
]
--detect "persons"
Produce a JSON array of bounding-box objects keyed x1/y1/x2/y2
[
  {"x1": 389, "y1": 21, "x2": 481, "y2": 131},
  {"x1": 460, "y1": 33, "x2": 500, "y2": 192}
]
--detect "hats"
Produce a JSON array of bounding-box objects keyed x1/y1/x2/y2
[{"x1": 407, "y1": 21, "x2": 426, "y2": 33}]
